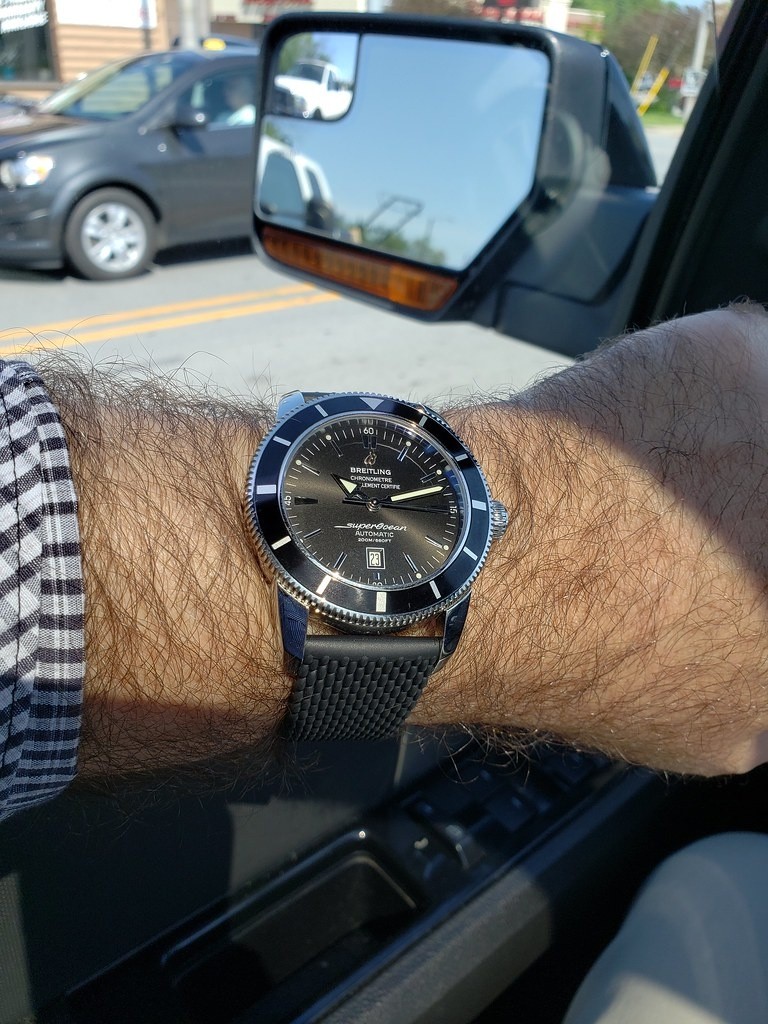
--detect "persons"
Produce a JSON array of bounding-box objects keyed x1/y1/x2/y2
[
  {"x1": 0, "y1": 298, "x2": 768, "y2": 1024},
  {"x1": 203, "y1": 73, "x2": 255, "y2": 130}
]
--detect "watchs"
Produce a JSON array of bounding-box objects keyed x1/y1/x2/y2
[{"x1": 240, "y1": 386, "x2": 509, "y2": 745}]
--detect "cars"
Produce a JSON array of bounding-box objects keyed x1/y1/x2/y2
[
  {"x1": 1, "y1": 49, "x2": 260, "y2": 281},
  {"x1": 276, "y1": 59, "x2": 353, "y2": 121},
  {"x1": 262, "y1": 141, "x2": 334, "y2": 232}
]
